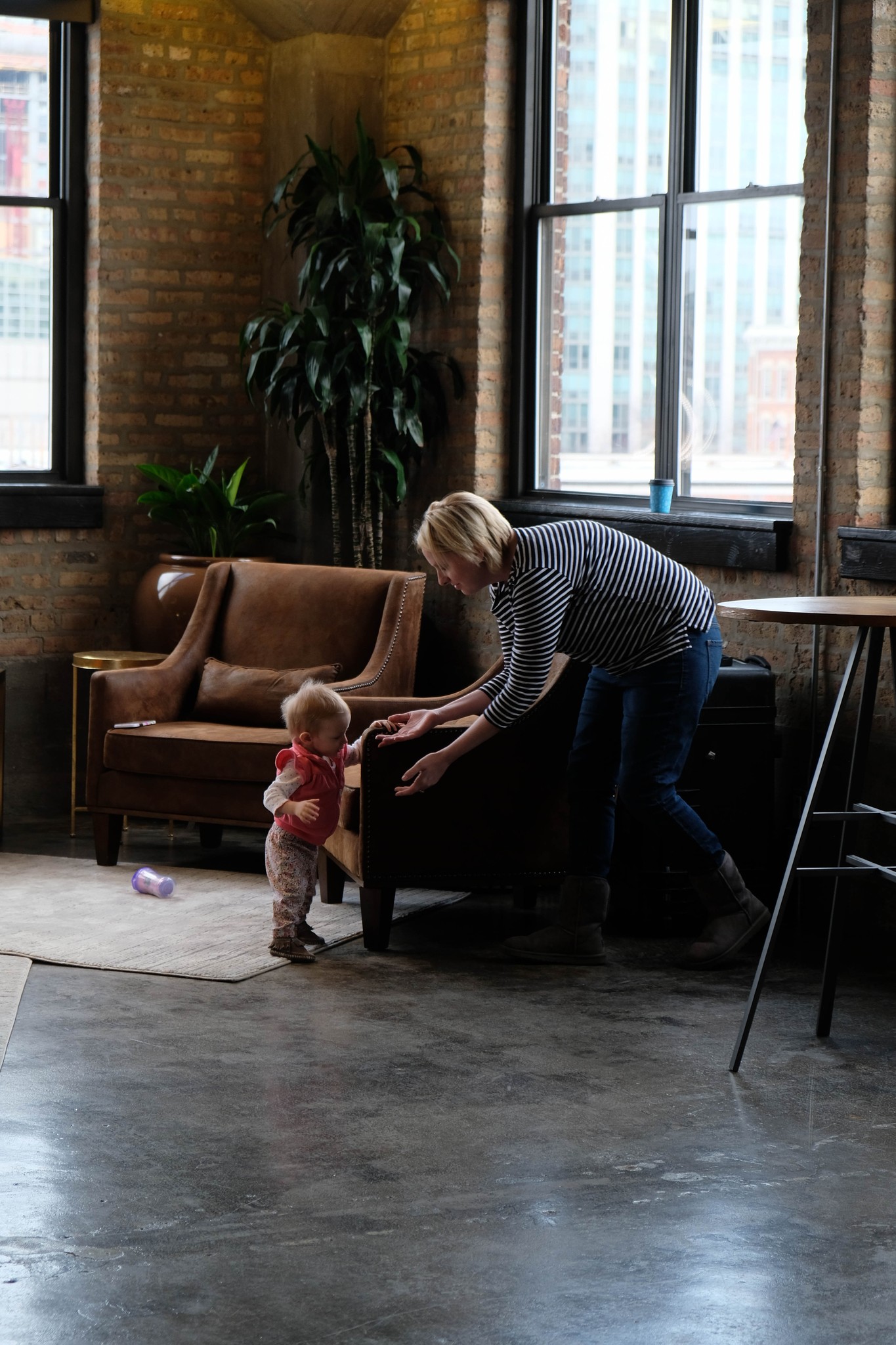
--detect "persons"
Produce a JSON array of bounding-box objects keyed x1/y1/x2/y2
[
  {"x1": 375, "y1": 491, "x2": 773, "y2": 968},
  {"x1": 264, "y1": 678, "x2": 399, "y2": 960}
]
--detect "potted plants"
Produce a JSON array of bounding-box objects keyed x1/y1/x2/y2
[{"x1": 127, "y1": 442, "x2": 287, "y2": 654}]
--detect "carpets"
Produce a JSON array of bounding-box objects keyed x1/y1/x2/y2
[
  {"x1": 0, "y1": 953, "x2": 33, "y2": 1073},
  {"x1": 0, "y1": 852, "x2": 472, "y2": 982}
]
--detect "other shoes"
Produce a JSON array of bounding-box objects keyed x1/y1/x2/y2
[
  {"x1": 295, "y1": 920, "x2": 325, "y2": 945},
  {"x1": 268, "y1": 937, "x2": 316, "y2": 962}
]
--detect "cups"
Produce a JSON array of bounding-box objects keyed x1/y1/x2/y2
[
  {"x1": 132, "y1": 866, "x2": 176, "y2": 900},
  {"x1": 649, "y1": 478, "x2": 675, "y2": 513}
]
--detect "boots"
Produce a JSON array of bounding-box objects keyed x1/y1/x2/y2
[
  {"x1": 685, "y1": 850, "x2": 772, "y2": 968},
  {"x1": 497, "y1": 877, "x2": 611, "y2": 966}
]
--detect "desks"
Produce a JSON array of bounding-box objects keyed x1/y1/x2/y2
[
  {"x1": 714, "y1": 596, "x2": 896, "y2": 1073},
  {"x1": 70, "y1": 651, "x2": 174, "y2": 847}
]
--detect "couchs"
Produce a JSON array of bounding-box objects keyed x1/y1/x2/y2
[
  {"x1": 316, "y1": 652, "x2": 593, "y2": 952},
  {"x1": 88, "y1": 560, "x2": 426, "y2": 867}
]
